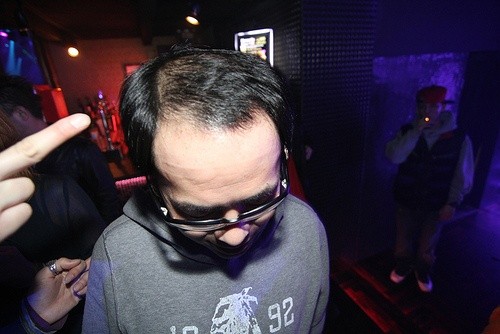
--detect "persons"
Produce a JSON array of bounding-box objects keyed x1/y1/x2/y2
[
  {"x1": 385, "y1": 86, "x2": 472, "y2": 292},
  {"x1": 81, "y1": 49, "x2": 329, "y2": 334},
  {"x1": 0, "y1": 76, "x2": 122, "y2": 334}
]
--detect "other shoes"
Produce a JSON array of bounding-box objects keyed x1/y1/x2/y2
[
  {"x1": 390, "y1": 265, "x2": 415, "y2": 283},
  {"x1": 413, "y1": 265, "x2": 433, "y2": 293}
]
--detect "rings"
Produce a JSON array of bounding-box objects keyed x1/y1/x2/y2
[{"x1": 46, "y1": 260, "x2": 60, "y2": 276}]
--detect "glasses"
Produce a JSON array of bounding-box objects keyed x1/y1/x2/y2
[{"x1": 145, "y1": 145, "x2": 297, "y2": 234}]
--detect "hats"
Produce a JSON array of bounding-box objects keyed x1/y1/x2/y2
[{"x1": 417, "y1": 85, "x2": 445, "y2": 105}]
